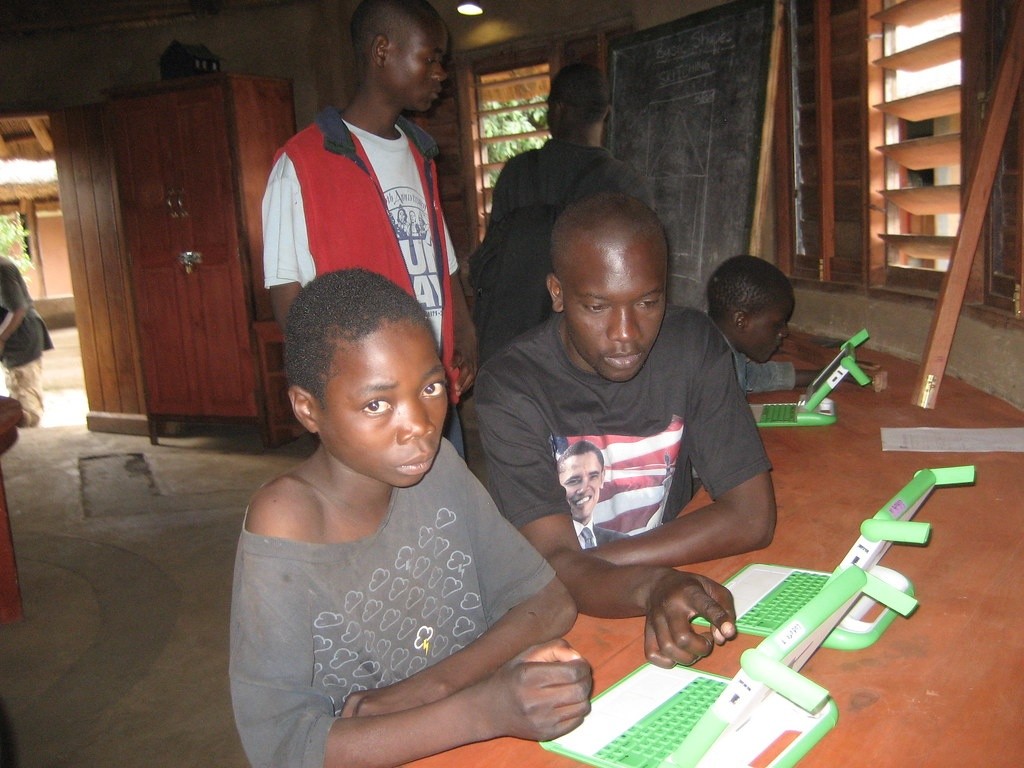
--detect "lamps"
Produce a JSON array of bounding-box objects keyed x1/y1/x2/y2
[{"x1": 457, "y1": 0, "x2": 485, "y2": 17}]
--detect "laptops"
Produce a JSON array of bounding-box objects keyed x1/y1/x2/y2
[
  {"x1": 750, "y1": 328, "x2": 872, "y2": 426},
  {"x1": 537, "y1": 561, "x2": 917, "y2": 768},
  {"x1": 695, "y1": 462, "x2": 977, "y2": 654}
]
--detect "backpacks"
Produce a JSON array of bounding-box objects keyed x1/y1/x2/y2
[{"x1": 466, "y1": 148, "x2": 630, "y2": 371}]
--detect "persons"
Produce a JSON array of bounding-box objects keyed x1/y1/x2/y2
[
  {"x1": 0, "y1": 256, "x2": 56, "y2": 427},
  {"x1": 229, "y1": 269, "x2": 592, "y2": 768},
  {"x1": 261, "y1": 0, "x2": 480, "y2": 464},
  {"x1": 474, "y1": 192, "x2": 777, "y2": 668},
  {"x1": 468, "y1": 64, "x2": 638, "y2": 362},
  {"x1": 706, "y1": 254, "x2": 881, "y2": 394},
  {"x1": 556, "y1": 441, "x2": 631, "y2": 549}
]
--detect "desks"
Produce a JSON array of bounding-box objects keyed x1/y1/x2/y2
[{"x1": 401, "y1": 334, "x2": 1024, "y2": 768}]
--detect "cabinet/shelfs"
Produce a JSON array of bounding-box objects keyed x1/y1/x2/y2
[{"x1": 101, "y1": 72, "x2": 298, "y2": 449}]
